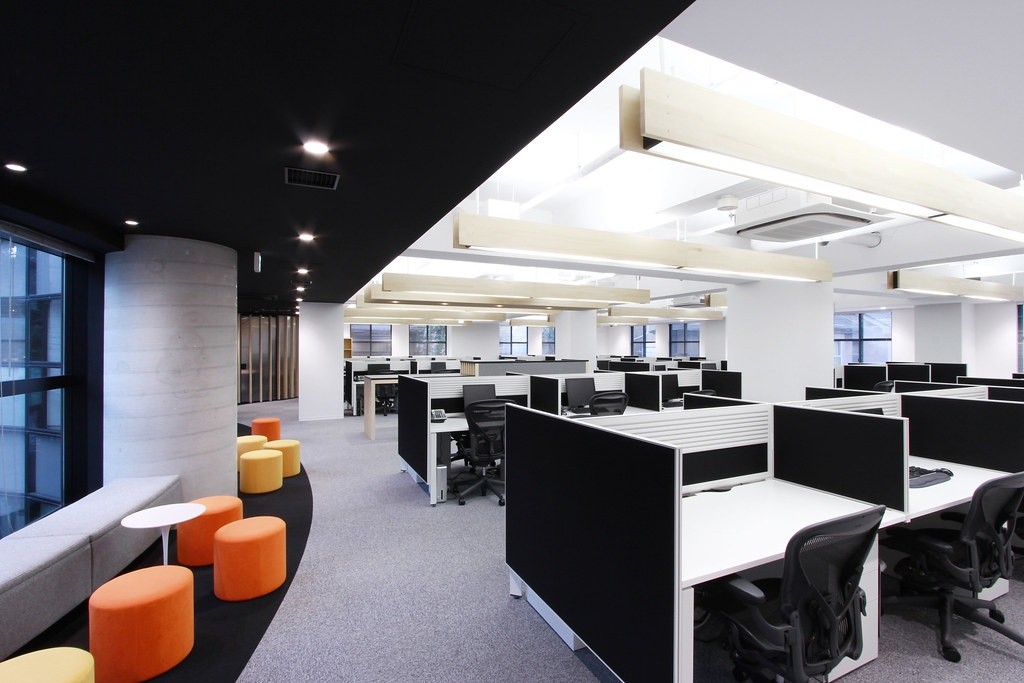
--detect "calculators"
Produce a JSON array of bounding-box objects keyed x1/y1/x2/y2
[{"x1": 432, "y1": 409, "x2": 447, "y2": 420}]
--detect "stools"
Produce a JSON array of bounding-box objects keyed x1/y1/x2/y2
[
  {"x1": 251, "y1": 418, "x2": 281, "y2": 442},
  {"x1": 89, "y1": 565, "x2": 194, "y2": 683},
  {"x1": 240, "y1": 450, "x2": 283, "y2": 493},
  {"x1": 0, "y1": 647, "x2": 95, "y2": 683},
  {"x1": 237, "y1": 435, "x2": 268, "y2": 472},
  {"x1": 262, "y1": 439, "x2": 301, "y2": 478},
  {"x1": 213, "y1": 516, "x2": 286, "y2": 602},
  {"x1": 176, "y1": 495, "x2": 243, "y2": 566}
]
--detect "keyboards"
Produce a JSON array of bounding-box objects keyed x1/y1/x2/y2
[
  {"x1": 572, "y1": 408, "x2": 590, "y2": 414},
  {"x1": 909, "y1": 465, "x2": 949, "y2": 488},
  {"x1": 662, "y1": 402, "x2": 683, "y2": 407}
]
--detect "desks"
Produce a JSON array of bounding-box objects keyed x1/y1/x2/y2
[
  {"x1": 120, "y1": 502, "x2": 207, "y2": 566},
  {"x1": 342, "y1": 355, "x2": 1024, "y2": 683}
]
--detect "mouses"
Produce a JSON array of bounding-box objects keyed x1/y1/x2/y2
[{"x1": 935, "y1": 468, "x2": 955, "y2": 477}]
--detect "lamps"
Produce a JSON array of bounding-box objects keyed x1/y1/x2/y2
[
  {"x1": 618, "y1": 65, "x2": 1024, "y2": 245},
  {"x1": 452, "y1": 186, "x2": 833, "y2": 284},
  {"x1": 886, "y1": 262, "x2": 1024, "y2": 302},
  {"x1": 717, "y1": 194, "x2": 739, "y2": 211},
  {"x1": 344, "y1": 257, "x2": 727, "y2": 327}
]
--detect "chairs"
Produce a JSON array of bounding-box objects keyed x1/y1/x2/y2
[
  {"x1": 375, "y1": 369, "x2": 397, "y2": 416},
  {"x1": 588, "y1": 392, "x2": 628, "y2": 416},
  {"x1": 872, "y1": 380, "x2": 894, "y2": 392},
  {"x1": 442, "y1": 399, "x2": 515, "y2": 506},
  {"x1": 694, "y1": 505, "x2": 886, "y2": 683},
  {"x1": 880, "y1": 471, "x2": 1024, "y2": 663}
]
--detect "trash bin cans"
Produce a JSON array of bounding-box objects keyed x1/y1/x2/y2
[{"x1": 429, "y1": 465, "x2": 447, "y2": 503}]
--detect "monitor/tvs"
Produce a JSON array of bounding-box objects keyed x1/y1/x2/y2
[
  {"x1": 565, "y1": 378, "x2": 595, "y2": 409},
  {"x1": 368, "y1": 364, "x2": 390, "y2": 375},
  {"x1": 637, "y1": 360, "x2": 644, "y2": 362},
  {"x1": 653, "y1": 364, "x2": 667, "y2": 371},
  {"x1": 431, "y1": 362, "x2": 446, "y2": 374},
  {"x1": 674, "y1": 359, "x2": 682, "y2": 362},
  {"x1": 661, "y1": 374, "x2": 679, "y2": 402},
  {"x1": 463, "y1": 384, "x2": 495, "y2": 408}
]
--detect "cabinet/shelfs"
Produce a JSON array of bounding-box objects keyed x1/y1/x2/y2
[{"x1": 344, "y1": 338, "x2": 352, "y2": 358}]
